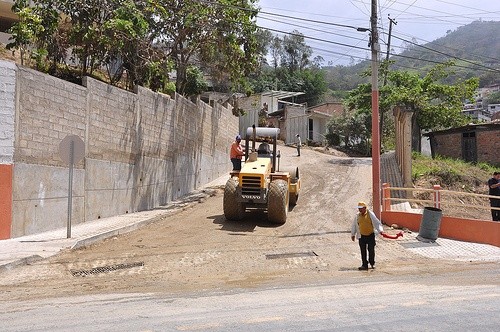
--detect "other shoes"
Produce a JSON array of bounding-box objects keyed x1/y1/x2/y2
[
  {"x1": 372, "y1": 264, "x2": 375, "y2": 268},
  {"x1": 358, "y1": 266, "x2": 368, "y2": 270}
]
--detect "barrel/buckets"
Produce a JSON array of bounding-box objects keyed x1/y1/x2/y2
[{"x1": 419, "y1": 207, "x2": 442, "y2": 240}]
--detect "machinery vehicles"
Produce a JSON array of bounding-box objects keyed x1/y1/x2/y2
[{"x1": 223, "y1": 123, "x2": 301, "y2": 225}]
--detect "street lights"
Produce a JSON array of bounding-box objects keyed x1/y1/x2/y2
[{"x1": 357, "y1": 26, "x2": 382, "y2": 225}]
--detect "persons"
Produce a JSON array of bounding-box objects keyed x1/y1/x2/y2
[
  {"x1": 296, "y1": 134, "x2": 301, "y2": 156},
  {"x1": 351, "y1": 202, "x2": 383, "y2": 270},
  {"x1": 259, "y1": 139, "x2": 270, "y2": 152},
  {"x1": 488, "y1": 172, "x2": 500, "y2": 222},
  {"x1": 230, "y1": 135, "x2": 244, "y2": 177}
]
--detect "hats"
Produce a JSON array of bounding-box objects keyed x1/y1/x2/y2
[
  {"x1": 236, "y1": 135, "x2": 242, "y2": 140},
  {"x1": 357, "y1": 202, "x2": 367, "y2": 209}
]
